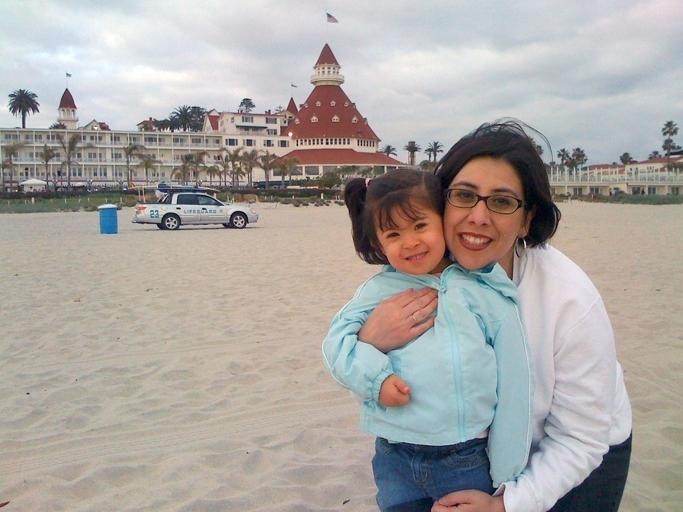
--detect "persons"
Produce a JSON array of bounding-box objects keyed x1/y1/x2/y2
[
  {"x1": 356, "y1": 116, "x2": 631, "y2": 510},
  {"x1": 321, "y1": 166, "x2": 533, "y2": 511}
]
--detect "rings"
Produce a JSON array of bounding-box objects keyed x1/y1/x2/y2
[{"x1": 411, "y1": 314, "x2": 417, "y2": 322}]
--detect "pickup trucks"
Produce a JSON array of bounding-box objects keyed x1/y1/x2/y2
[{"x1": 131, "y1": 193, "x2": 258, "y2": 230}]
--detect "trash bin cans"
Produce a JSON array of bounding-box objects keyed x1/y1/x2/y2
[{"x1": 98, "y1": 204, "x2": 118, "y2": 234}]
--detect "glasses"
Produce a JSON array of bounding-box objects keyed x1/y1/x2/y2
[{"x1": 442, "y1": 188, "x2": 529, "y2": 215}]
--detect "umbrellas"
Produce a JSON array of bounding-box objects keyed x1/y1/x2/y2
[{"x1": 20, "y1": 177, "x2": 48, "y2": 188}]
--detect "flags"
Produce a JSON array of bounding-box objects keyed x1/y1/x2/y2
[
  {"x1": 326, "y1": 13, "x2": 339, "y2": 24},
  {"x1": 67, "y1": 73, "x2": 72, "y2": 77}
]
